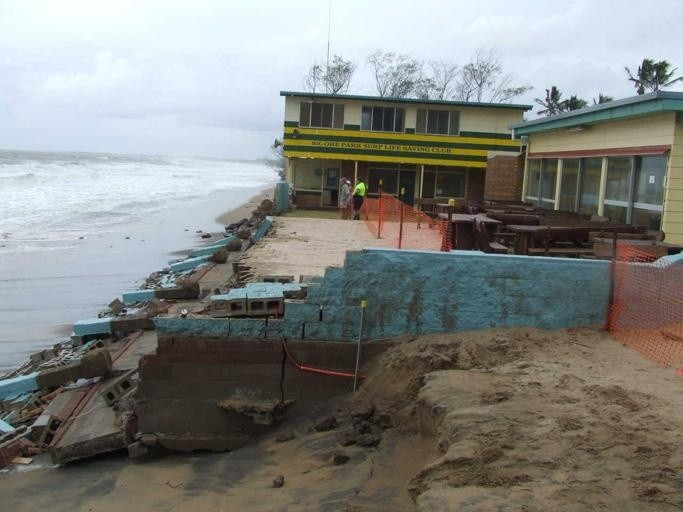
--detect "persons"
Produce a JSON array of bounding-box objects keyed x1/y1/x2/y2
[{"x1": 340, "y1": 177, "x2": 366, "y2": 220}]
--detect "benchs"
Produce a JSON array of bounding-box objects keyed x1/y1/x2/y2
[{"x1": 415, "y1": 197, "x2": 683, "y2": 257}]
